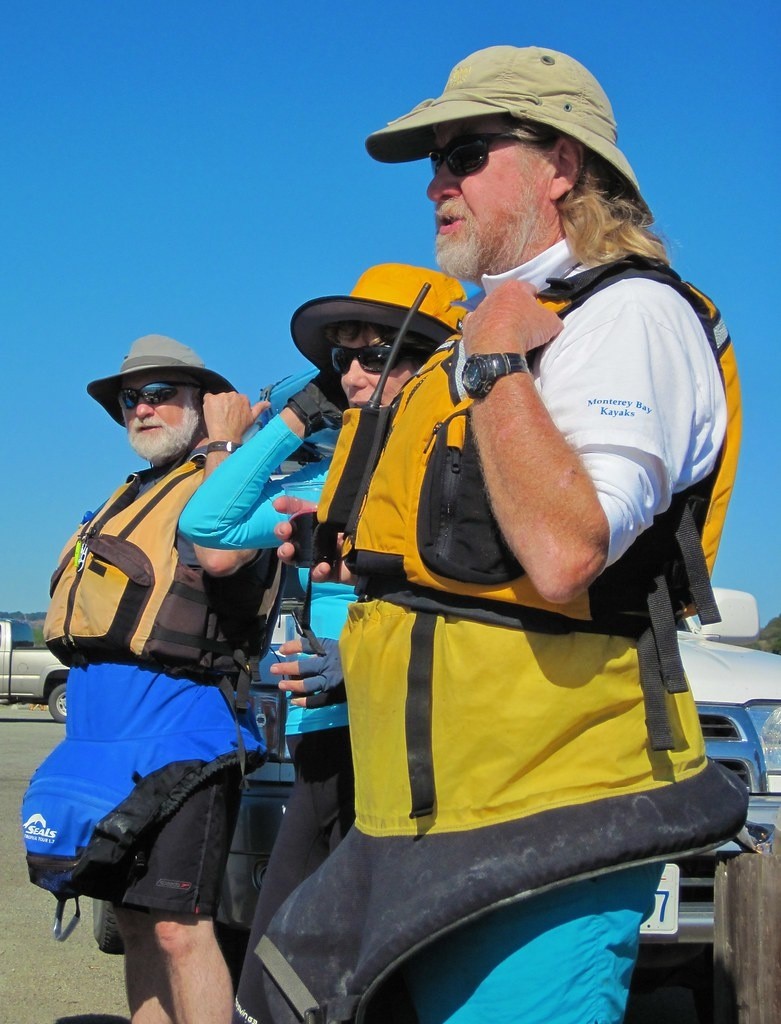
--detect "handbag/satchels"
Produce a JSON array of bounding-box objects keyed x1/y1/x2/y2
[{"x1": 23, "y1": 729, "x2": 270, "y2": 898}]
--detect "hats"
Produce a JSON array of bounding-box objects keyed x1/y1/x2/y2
[
  {"x1": 291, "y1": 262, "x2": 471, "y2": 370},
  {"x1": 365, "y1": 42, "x2": 654, "y2": 222},
  {"x1": 86, "y1": 333, "x2": 241, "y2": 426}
]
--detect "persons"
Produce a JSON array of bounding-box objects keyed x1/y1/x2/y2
[
  {"x1": 176, "y1": 263, "x2": 471, "y2": 1024},
  {"x1": 42, "y1": 334, "x2": 281, "y2": 1024},
  {"x1": 273, "y1": 45, "x2": 745, "y2": 1024}
]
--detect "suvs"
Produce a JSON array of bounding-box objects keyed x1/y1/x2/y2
[{"x1": 92, "y1": 590, "x2": 781, "y2": 956}]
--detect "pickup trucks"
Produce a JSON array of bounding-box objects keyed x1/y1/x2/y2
[{"x1": 1, "y1": 619, "x2": 70, "y2": 724}]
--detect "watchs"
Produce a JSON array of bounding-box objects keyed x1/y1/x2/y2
[{"x1": 461, "y1": 352, "x2": 529, "y2": 399}]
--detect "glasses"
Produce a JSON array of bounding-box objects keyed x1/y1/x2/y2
[
  {"x1": 116, "y1": 381, "x2": 207, "y2": 410},
  {"x1": 329, "y1": 344, "x2": 417, "y2": 374},
  {"x1": 427, "y1": 133, "x2": 562, "y2": 178}
]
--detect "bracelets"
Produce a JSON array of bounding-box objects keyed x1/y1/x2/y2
[{"x1": 206, "y1": 441, "x2": 242, "y2": 453}]
